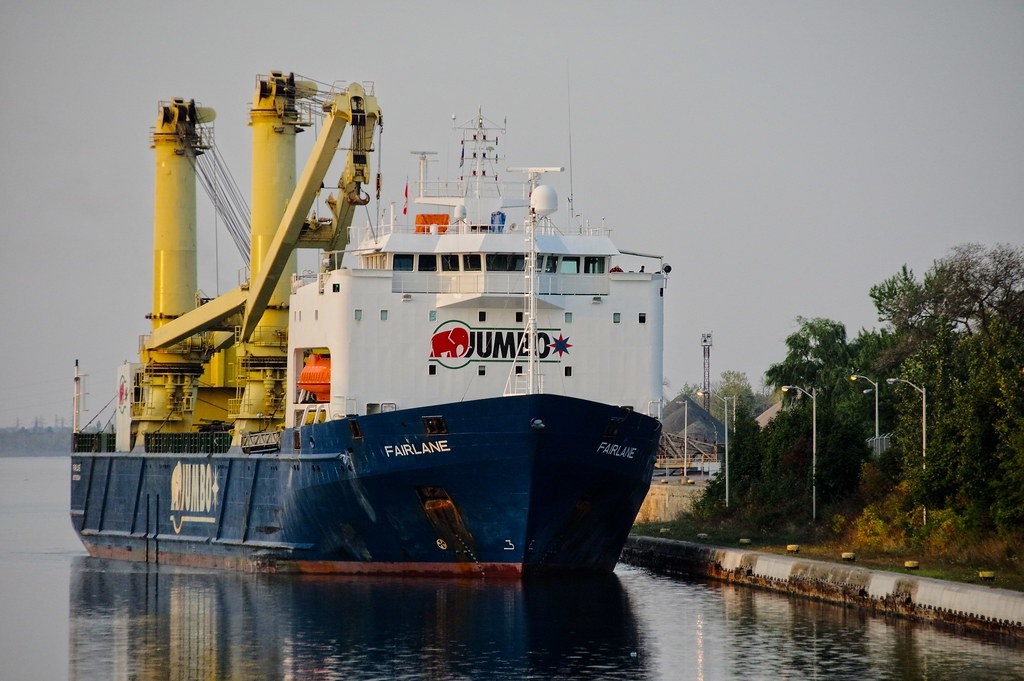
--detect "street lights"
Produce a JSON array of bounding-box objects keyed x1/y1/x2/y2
[
  {"x1": 698, "y1": 391, "x2": 729, "y2": 508},
  {"x1": 675, "y1": 400, "x2": 687, "y2": 477},
  {"x1": 886, "y1": 377, "x2": 925, "y2": 524},
  {"x1": 850, "y1": 375, "x2": 879, "y2": 438},
  {"x1": 782, "y1": 384, "x2": 816, "y2": 522},
  {"x1": 723, "y1": 396, "x2": 736, "y2": 435}
]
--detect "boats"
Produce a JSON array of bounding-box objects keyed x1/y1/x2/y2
[{"x1": 67, "y1": 67, "x2": 672, "y2": 587}]
